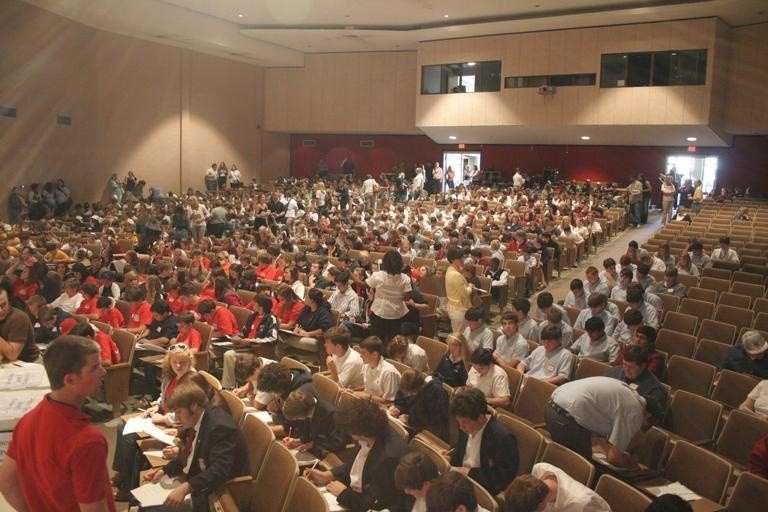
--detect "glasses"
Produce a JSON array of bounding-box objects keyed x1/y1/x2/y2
[{"x1": 169, "y1": 344, "x2": 187, "y2": 350}]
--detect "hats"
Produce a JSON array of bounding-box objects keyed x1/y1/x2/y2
[
  {"x1": 741, "y1": 330, "x2": 768, "y2": 355},
  {"x1": 59, "y1": 318, "x2": 78, "y2": 336}
]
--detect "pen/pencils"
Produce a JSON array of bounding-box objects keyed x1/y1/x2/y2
[
  {"x1": 137, "y1": 407, "x2": 146, "y2": 412},
  {"x1": 13, "y1": 364, "x2": 22, "y2": 367},
  {"x1": 289, "y1": 424, "x2": 293, "y2": 437},
  {"x1": 142, "y1": 468, "x2": 161, "y2": 483},
  {"x1": 306, "y1": 460, "x2": 319, "y2": 478},
  {"x1": 138, "y1": 330, "x2": 144, "y2": 337}
]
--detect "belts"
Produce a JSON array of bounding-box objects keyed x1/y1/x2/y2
[{"x1": 547, "y1": 398, "x2": 574, "y2": 421}]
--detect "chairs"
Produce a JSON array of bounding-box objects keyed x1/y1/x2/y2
[{"x1": 0, "y1": 183, "x2": 768, "y2": 511}]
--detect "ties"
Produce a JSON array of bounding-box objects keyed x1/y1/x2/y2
[{"x1": 181, "y1": 434, "x2": 193, "y2": 468}]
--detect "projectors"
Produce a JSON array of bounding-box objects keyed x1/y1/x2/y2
[{"x1": 539, "y1": 85, "x2": 553, "y2": 92}]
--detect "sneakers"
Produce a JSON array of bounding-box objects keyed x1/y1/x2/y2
[
  {"x1": 660, "y1": 223, "x2": 665, "y2": 229},
  {"x1": 111, "y1": 472, "x2": 134, "y2": 501},
  {"x1": 629, "y1": 223, "x2": 640, "y2": 229}
]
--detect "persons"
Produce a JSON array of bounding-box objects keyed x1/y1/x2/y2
[
  {"x1": 0, "y1": 162, "x2": 651, "y2": 363},
  {"x1": 110, "y1": 311, "x2": 694, "y2": 512},
  {"x1": 660, "y1": 167, "x2": 751, "y2": 229},
  {"x1": 642, "y1": 236, "x2": 741, "y2": 299},
  {"x1": 1, "y1": 335, "x2": 117, "y2": 512},
  {"x1": 721, "y1": 331, "x2": 768, "y2": 419},
  {"x1": 750, "y1": 435, "x2": 768, "y2": 480}
]
